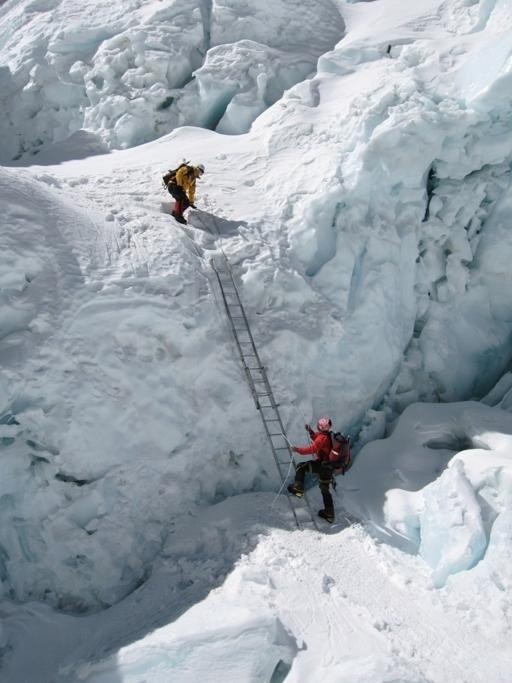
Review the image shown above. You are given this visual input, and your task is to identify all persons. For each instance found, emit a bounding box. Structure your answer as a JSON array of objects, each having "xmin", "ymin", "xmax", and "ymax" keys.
[
  {"xmin": 287, "ymin": 417, "xmax": 335, "ymax": 523},
  {"xmin": 168, "ymin": 163, "xmax": 205, "ymax": 225}
]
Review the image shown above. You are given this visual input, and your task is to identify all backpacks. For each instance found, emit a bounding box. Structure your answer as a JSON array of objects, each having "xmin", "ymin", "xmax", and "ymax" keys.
[
  {"xmin": 163, "ymin": 163, "xmax": 192, "ymax": 185},
  {"xmin": 312, "ymin": 432, "xmax": 350, "ymax": 476}
]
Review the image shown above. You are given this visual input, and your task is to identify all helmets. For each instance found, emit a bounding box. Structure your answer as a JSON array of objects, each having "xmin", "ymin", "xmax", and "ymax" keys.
[
  {"xmin": 317, "ymin": 418, "xmax": 332, "ymax": 431},
  {"xmin": 198, "ymin": 164, "xmax": 205, "ymax": 170}
]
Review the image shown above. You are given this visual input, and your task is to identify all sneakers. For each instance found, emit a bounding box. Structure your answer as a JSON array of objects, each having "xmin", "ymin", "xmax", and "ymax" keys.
[
  {"xmin": 171, "ymin": 210, "xmax": 187, "ymax": 225},
  {"xmin": 319, "ymin": 510, "xmax": 334, "ymax": 523},
  {"xmin": 288, "ymin": 485, "xmax": 304, "ymax": 498}
]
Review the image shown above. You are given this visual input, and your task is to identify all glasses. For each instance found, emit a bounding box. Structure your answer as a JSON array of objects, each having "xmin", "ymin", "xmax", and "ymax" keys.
[{"xmin": 198, "ymin": 167, "xmax": 204, "ymax": 173}]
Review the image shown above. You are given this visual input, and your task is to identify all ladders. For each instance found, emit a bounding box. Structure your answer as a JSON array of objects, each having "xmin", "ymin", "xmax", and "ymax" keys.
[{"xmin": 204, "ymin": 252, "xmax": 321, "ymax": 533}]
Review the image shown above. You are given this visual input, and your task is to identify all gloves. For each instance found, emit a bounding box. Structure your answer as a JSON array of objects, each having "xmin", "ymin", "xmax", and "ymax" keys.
[
  {"xmin": 189, "ymin": 202, "xmax": 194, "ymax": 206},
  {"xmin": 292, "ymin": 447, "xmax": 296, "ymax": 451},
  {"xmin": 177, "ymin": 186, "xmax": 183, "ymax": 190},
  {"xmin": 305, "ymin": 424, "xmax": 311, "ymax": 430}
]
